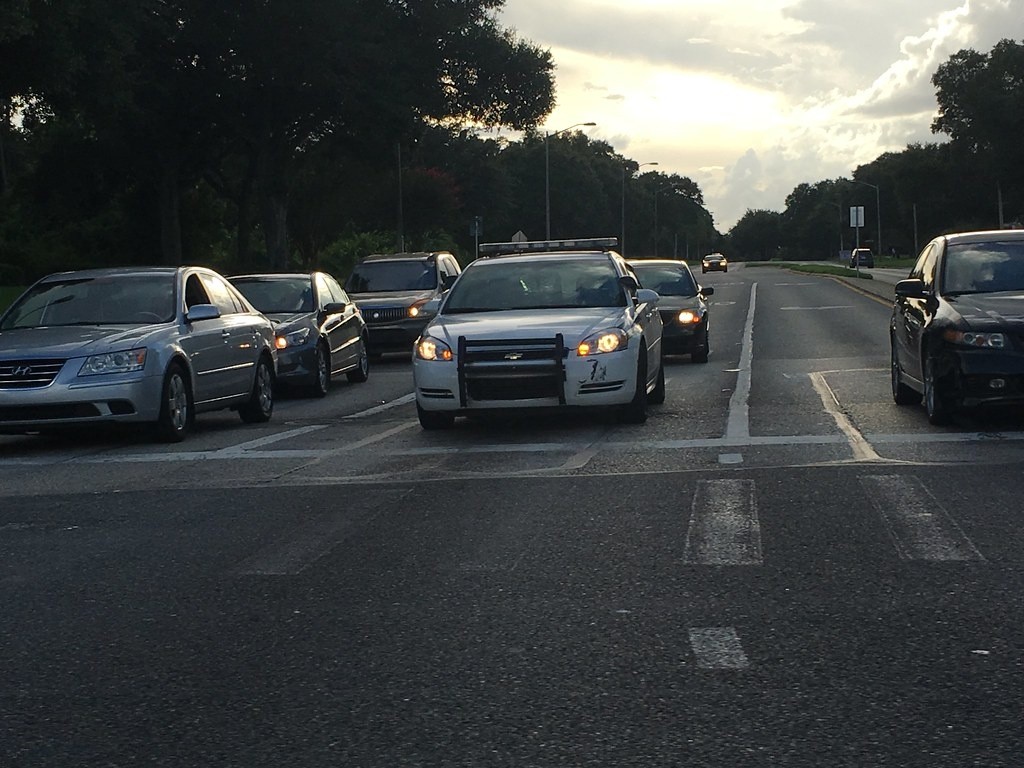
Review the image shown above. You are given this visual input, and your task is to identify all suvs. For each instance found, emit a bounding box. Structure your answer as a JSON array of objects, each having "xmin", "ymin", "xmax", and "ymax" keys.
[{"xmin": 342, "ymin": 251, "xmax": 461, "ymax": 364}]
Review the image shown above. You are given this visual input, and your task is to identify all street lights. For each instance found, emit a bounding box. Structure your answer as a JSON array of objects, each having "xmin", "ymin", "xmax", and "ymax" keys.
[
  {"xmin": 849, "ymin": 180, "xmax": 881, "ymax": 260},
  {"xmin": 621, "ymin": 162, "xmax": 658, "ymax": 255},
  {"xmin": 544, "ymin": 123, "xmax": 597, "ymax": 246}
]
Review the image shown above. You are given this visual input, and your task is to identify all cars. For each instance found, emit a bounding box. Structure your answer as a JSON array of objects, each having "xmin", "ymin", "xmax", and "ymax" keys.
[
  {"xmin": 223, "ymin": 272, "xmax": 370, "ymax": 399},
  {"xmin": 890, "ymin": 229, "xmax": 1024, "ymax": 432},
  {"xmin": 850, "ymin": 248, "xmax": 874, "ymax": 268},
  {"xmin": 702, "ymin": 253, "xmax": 728, "ymax": 273},
  {"xmin": 412, "ymin": 237, "xmax": 664, "ymax": 432},
  {"xmin": 626, "ymin": 259, "xmax": 715, "ymax": 363},
  {"xmin": 0, "ymin": 267, "xmax": 279, "ymax": 457}
]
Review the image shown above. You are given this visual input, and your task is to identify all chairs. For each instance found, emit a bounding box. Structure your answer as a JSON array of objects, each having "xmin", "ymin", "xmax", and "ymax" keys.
[{"xmin": 486, "ymin": 276, "xmax": 530, "ymax": 308}]
